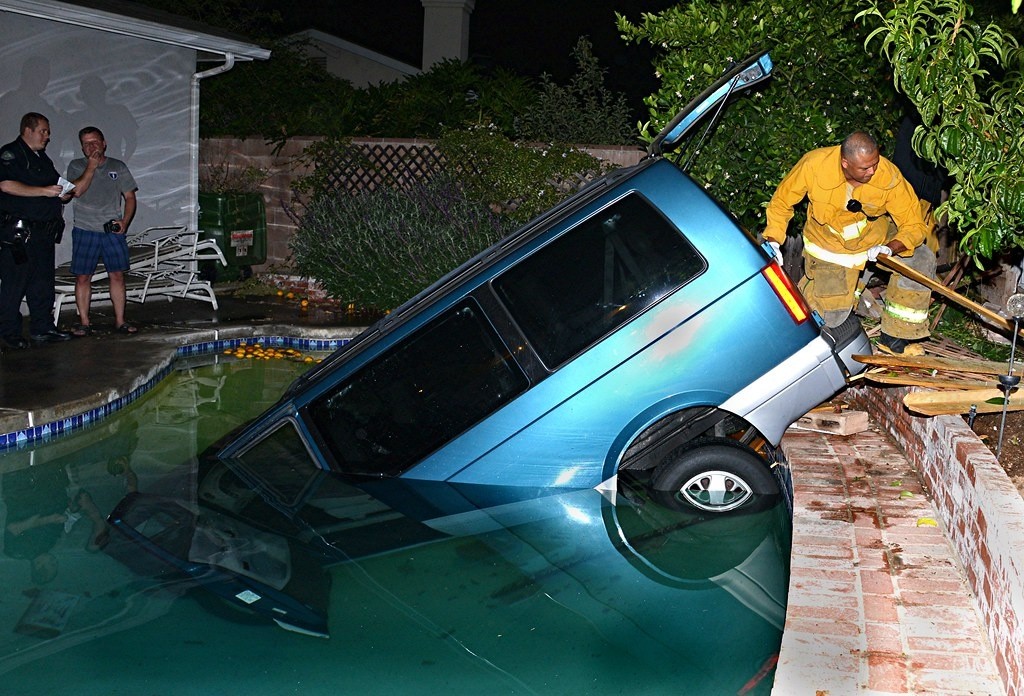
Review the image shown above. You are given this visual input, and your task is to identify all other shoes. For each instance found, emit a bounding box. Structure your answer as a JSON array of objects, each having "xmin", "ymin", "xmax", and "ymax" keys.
[{"xmin": 874, "ymin": 331, "xmax": 925, "ymax": 360}]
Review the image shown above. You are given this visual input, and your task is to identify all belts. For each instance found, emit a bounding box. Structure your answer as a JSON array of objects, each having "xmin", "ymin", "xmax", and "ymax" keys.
[{"xmin": 0, "ymin": 210, "xmax": 46, "ymax": 231}]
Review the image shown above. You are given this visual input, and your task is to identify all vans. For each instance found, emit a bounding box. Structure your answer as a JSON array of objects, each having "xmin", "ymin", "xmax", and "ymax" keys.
[{"xmin": 105, "ymin": 44, "xmax": 872, "ymax": 639}]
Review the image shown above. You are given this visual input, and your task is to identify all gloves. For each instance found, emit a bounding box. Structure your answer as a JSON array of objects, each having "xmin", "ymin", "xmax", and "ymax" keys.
[
  {"xmin": 867, "ymin": 246, "xmax": 892, "ymax": 262},
  {"xmin": 769, "ymin": 241, "xmax": 784, "ymax": 267}
]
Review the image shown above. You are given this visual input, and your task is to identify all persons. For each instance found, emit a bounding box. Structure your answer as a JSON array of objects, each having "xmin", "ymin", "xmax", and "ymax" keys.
[
  {"xmin": 0, "ymin": 113, "xmax": 73, "ymax": 347},
  {"xmin": 762, "ymin": 115, "xmax": 957, "ymax": 351},
  {"xmin": 66, "ymin": 126, "xmax": 138, "ymax": 335}
]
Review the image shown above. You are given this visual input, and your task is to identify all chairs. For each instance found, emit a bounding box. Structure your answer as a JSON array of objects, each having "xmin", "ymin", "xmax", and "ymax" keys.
[{"xmin": 22, "ymin": 224, "xmax": 227, "ymax": 326}]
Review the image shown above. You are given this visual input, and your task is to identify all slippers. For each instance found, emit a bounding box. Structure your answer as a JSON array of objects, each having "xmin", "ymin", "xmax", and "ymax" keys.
[
  {"xmin": 74, "ymin": 325, "xmax": 92, "ymax": 337},
  {"xmin": 114, "ymin": 323, "xmax": 138, "ymax": 334}
]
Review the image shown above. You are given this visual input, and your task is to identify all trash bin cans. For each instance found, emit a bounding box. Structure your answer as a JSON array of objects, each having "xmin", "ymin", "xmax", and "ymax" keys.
[{"xmin": 195, "ymin": 189, "xmax": 267, "ymax": 286}]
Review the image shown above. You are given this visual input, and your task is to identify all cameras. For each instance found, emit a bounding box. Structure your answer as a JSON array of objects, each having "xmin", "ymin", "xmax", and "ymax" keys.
[{"xmin": 103, "ymin": 219, "xmax": 121, "ymax": 233}]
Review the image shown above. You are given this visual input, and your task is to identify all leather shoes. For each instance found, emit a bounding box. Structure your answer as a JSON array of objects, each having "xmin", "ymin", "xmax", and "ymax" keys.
[
  {"xmin": 30, "ymin": 325, "xmax": 73, "ymax": 340},
  {"xmin": 0, "ymin": 334, "xmax": 28, "ymax": 349}
]
